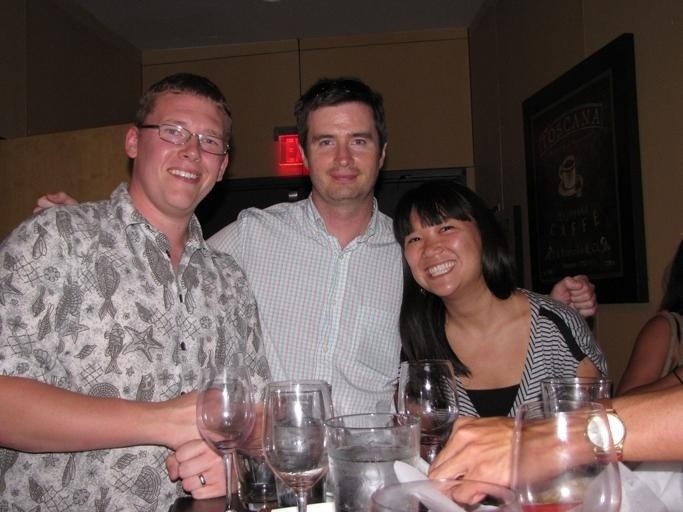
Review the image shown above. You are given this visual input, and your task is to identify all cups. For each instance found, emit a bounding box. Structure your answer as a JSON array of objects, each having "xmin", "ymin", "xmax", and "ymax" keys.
[
  {"xmin": 396, "ymin": 357, "xmax": 459, "ymax": 466},
  {"xmin": 508, "ymin": 402, "xmax": 622, "ymax": 512},
  {"xmin": 322, "ymin": 412, "xmax": 421, "ymax": 512},
  {"xmin": 542, "ymin": 377, "xmax": 614, "ymax": 419},
  {"xmin": 370, "ymin": 477, "xmax": 520, "ymax": 512},
  {"xmin": 193, "ymin": 364, "xmax": 255, "ymax": 511},
  {"xmin": 261, "ymin": 379, "xmax": 333, "ymax": 512},
  {"xmin": 232, "ymin": 445, "xmax": 290, "ymax": 512}
]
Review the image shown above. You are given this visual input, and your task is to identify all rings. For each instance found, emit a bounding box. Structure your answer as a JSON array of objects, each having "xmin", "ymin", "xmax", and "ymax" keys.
[{"xmin": 197, "ymin": 473, "xmax": 207, "ymax": 485}]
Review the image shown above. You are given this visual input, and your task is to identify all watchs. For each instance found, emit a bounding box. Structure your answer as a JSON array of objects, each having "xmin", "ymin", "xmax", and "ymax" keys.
[{"xmin": 586, "ymin": 397, "xmax": 626, "ymax": 464}]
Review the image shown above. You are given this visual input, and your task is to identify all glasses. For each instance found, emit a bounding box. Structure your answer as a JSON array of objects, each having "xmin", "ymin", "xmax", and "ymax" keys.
[{"xmin": 135, "ymin": 124, "xmax": 228, "ymax": 155}]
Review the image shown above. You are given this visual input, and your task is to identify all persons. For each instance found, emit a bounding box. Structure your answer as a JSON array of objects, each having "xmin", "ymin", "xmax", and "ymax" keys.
[
  {"xmin": 32, "ymin": 77, "xmax": 598, "ymax": 429},
  {"xmin": 427, "ymin": 360, "xmax": 683, "ymax": 512},
  {"xmin": 0, "ymin": 72, "xmax": 276, "ymax": 512},
  {"xmin": 393, "ymin": 178, "xmax": 612, "ymax": 451},
  {"xmin": 618, "ymin": 237, "xmax": 683, "ymax": 392}
]
